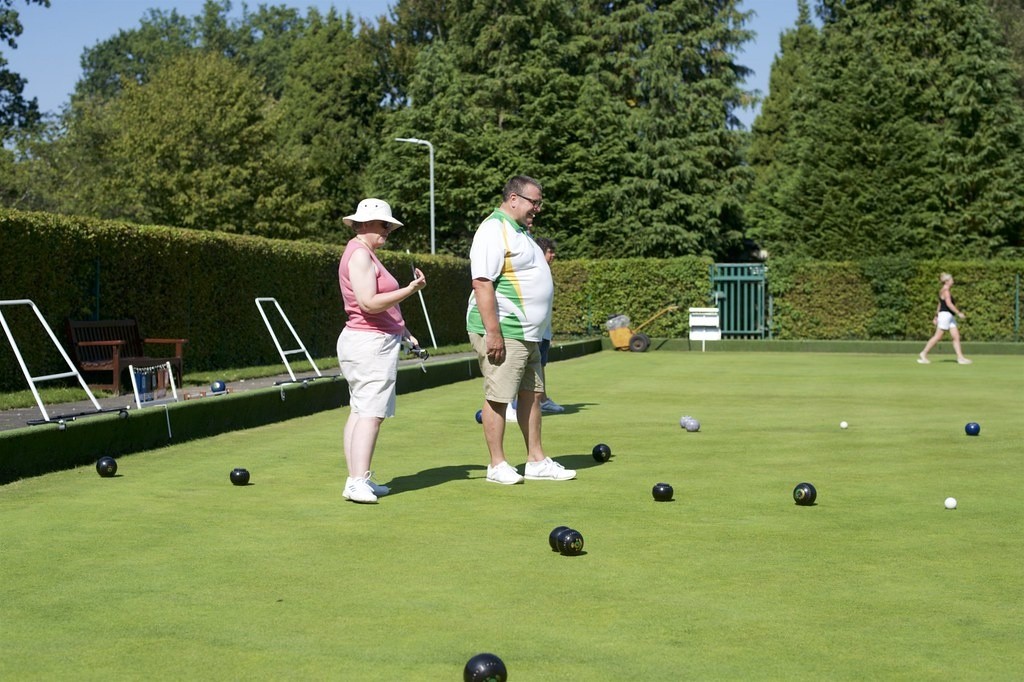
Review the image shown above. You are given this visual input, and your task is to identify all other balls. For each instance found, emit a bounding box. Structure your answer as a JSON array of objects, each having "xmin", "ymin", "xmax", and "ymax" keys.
[
  {"xmin": 840, "ymin": 421, "xmax": 849, "ymax": 428},
  {"xmin": 652, "ymin": 483, "xmax": 674, "ymax": 502},
  {"xmin": 96, "ymin": 457, "xmax": 117, "ymax": 477},
  {"xmin": 592, "ymin": 444, "xmax": 611, "ymax": 463},
  {"xmin": 549, "ymin": 526, "xmax": 570, "ymax": 550},
  {"xmin": 211, "ymin": 381, "xmax": 226, "ymax": 393},
  {"xmin": 230, "ymin": 468, "xmax": 250, "ymax": 486},
  {"xmin": 556, "ymin": 529, "xmax": 585, "ymax": 557},
  {"xmin": 944, "ymin": 497, "xmax": 957, "ymax": 509},
  {"xmin": 685, "ymin": 419, "xmax": 700, "ymax": 431},
  {"xmin": 966, "ymin": 422, "xmax": 980, "ymax": 435},
  {"xmin": 793, "ymin": 482, "xmax": 817, "ymax": 506},
  {"xmin": 475, "ymin": 410, "xmax": 483, "ymax": 424},
  {"xmin": 463, "ymin": 653, "xmax": 507, "ymax": 682},
  {"xmin": 680, "ymin": 416, "xmax": 693, "ymax": 427}
]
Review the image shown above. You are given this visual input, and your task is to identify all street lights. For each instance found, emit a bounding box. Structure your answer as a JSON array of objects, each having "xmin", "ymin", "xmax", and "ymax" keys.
[{"xmin": 393, "ymin": 138, "xmax": 436, "ymax": 257}]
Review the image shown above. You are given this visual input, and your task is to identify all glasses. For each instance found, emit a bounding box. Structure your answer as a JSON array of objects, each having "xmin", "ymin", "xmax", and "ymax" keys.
[
  {"xmin": 373, "ymin": 221, "xmax": 392, "ymax": 229},
  {"xmin": 517, "ymin": 194, "xmax": 544, "ymax": 207}
]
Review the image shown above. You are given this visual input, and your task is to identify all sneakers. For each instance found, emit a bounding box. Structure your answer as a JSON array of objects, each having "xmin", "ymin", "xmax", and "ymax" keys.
[
  {"xmin": 485, "ymin": 461, "xmax": 524, "ymax": 484},
  {"xmin": 523, "ymin": 456, "xmax": 577, "ymax": 481},
  {"xmin": 342, "ymin": 471, "xmax": 389, "ymax": 503}
]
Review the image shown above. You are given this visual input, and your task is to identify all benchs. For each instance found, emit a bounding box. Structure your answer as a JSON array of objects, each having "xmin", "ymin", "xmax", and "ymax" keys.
[{"xmin": 66, "ymin": 318, "xmax": 188, "ymax": 396}]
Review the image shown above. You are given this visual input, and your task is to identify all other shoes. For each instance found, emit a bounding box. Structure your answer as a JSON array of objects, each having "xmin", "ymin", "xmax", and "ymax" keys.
[
  {"xmin": 958, "ymin": 358, "xmax": 972, "ymax": 364},
  {"xmin": 540, "ymin": 398, "xmax": 564, "ymax": 413},
  {"xmin": 506, "ymin": 401, "xmax": 517, "ymax": 423},
  {"xmin": 917, "ymin": 359, "xmax": 931, "ymax": 364}
]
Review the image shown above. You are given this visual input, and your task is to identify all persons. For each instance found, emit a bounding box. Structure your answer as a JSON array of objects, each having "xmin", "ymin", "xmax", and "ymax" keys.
[
  {"xmin": 465, "ymin": 174, "xmax": 576, "ymax": 485},
  {"xmin": 335, "ymin": 198, "xmax": 426, "ymax": 504},
  {"xmin": 503, "ymin": 214, "xmax": 565, "ymax": 423},
  {"xmin": 916, "ymin": 271, "xmax": 973, "ymax": 364}
]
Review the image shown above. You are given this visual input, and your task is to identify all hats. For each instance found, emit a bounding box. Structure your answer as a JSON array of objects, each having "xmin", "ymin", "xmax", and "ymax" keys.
[{"xmin": 341, "ymin": 198, "xmax": 404, "ymax": 231}]
